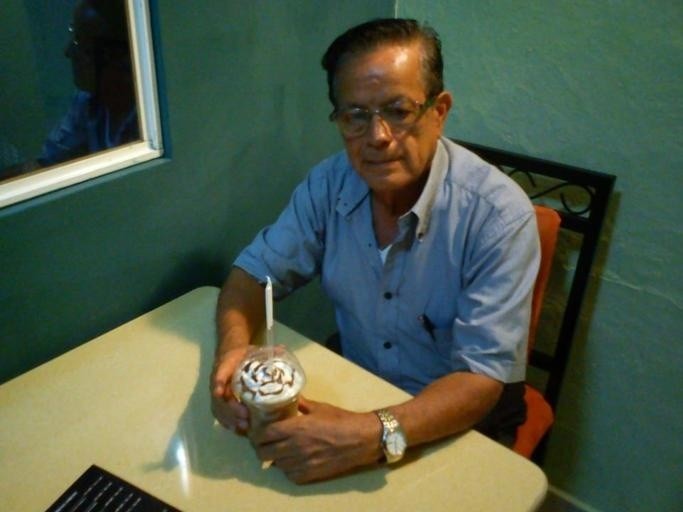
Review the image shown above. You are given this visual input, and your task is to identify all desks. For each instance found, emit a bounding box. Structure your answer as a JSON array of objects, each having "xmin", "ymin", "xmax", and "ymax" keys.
[{"xmin": 2, "ymin": 280, "xmax": 548, "ymax": 511}]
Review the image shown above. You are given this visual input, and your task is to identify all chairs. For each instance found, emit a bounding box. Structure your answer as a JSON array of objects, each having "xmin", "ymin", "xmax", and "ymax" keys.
[{"xmin": 439, "ymin": 141, "xmax": 618, "ymax": 470}]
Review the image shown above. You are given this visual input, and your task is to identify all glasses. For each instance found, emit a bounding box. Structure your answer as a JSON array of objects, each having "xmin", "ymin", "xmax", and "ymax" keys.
[{"xmin": 327, "ymin": 96, "xmax": 434, "ymax": 139}]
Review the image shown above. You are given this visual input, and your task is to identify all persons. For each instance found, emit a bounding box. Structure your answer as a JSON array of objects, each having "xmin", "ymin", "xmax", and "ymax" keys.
[
  {"xmin": 210, "ymin": 19, "xmax": 543, "ymax": 486},
  {"xmin": 20, "ymin": 0, "xmax": 143, "ymax": 180}
]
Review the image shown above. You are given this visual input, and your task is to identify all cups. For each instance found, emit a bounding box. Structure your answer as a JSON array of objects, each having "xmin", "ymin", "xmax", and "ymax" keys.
[{"xmin": 231, "ymin": 346, "xmax": 305, "ymax": 459}]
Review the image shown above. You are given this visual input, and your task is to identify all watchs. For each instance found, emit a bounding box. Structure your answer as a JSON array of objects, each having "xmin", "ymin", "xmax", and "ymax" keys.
[{"xmin": 375, "ymin": 409, "xmax": 406, "ymax": 467}]
